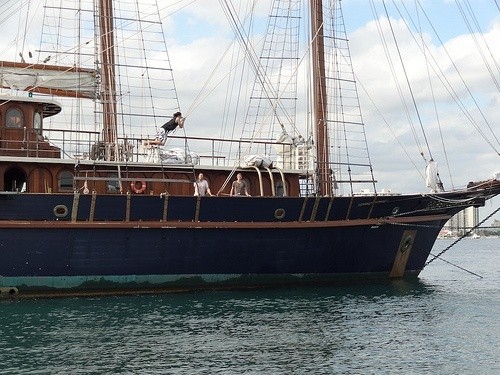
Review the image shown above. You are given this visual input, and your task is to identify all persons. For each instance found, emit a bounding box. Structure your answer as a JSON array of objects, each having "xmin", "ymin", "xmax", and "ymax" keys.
[
  {"xmin": 145, "ymin": 112, "xmax": 186, "ymax": 147},
  {"xmin": 230, "ymin": 173, "xmax": 251, "ymax": 197},
  {"xmin": 194, "ymin": 173, "xmax": 215, "ymax": 196}
]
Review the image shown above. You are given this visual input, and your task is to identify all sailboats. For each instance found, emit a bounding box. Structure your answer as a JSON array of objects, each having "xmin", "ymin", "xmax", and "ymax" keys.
[{"xmin": 0, "ymin": 0, "xmax": 499, "ymax": 299}]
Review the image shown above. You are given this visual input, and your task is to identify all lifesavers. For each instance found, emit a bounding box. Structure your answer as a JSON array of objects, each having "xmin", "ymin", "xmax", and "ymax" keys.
[{"xmin": 130, "ymin": 178, "xmax": 147, "ymax": 194}]
